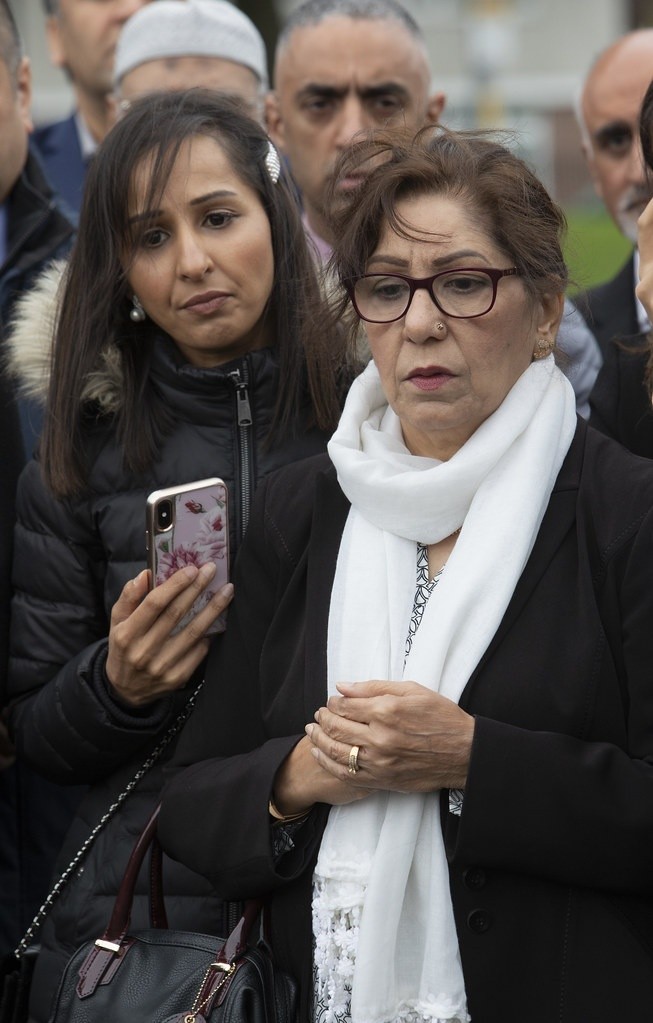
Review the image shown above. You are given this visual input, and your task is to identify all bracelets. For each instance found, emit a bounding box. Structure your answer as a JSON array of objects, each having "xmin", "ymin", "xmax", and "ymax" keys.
[{"xmin": 269, "ymin": 794, "xmax": 312, "ymax": 822}]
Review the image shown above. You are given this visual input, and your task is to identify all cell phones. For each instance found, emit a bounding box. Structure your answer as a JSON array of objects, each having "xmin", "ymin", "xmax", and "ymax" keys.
[{"xmin": 145, "ymin": 477, "xmax": 227, "ymax": 634}]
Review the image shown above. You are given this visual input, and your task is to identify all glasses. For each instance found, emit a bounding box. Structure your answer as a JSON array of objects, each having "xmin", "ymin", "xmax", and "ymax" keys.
[{"xmin": 342, "ymin": 268, "xmax": 545, "ymax": 324}]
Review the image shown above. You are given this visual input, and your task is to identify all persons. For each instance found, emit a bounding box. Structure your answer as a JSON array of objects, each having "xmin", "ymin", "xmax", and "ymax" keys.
[
  {"xmin": 0, "ymin": 0, "xmax": 653, "ymax": 690},
  {"xmin": 0, "ymin": 87, "xmax": 366, "ymax": 1023},
  {"xmin": 156, "ymin": 122, "xmax": 653, "ymax": 1023}
]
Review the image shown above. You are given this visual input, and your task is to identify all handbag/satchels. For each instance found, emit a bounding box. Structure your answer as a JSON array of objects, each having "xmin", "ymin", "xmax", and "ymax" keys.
[
  {"xmin": 0, "ymin": 951, "xmax": 42, "ymax": 1023},
  {"xmin": 48, "ymin": 804, "xmax": 296, "ymax": 1023}
]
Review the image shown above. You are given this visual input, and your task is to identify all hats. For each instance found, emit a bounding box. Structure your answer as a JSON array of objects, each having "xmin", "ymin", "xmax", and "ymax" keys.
[{"xmin": 110, "ymin": 0, "xmax": 267, "ymax": 96}]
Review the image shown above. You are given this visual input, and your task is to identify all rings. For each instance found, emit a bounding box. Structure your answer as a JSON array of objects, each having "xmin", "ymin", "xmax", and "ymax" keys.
[{"xmin": 349, "ymin": 746, "xmax": 361, "ymax": 774}]
